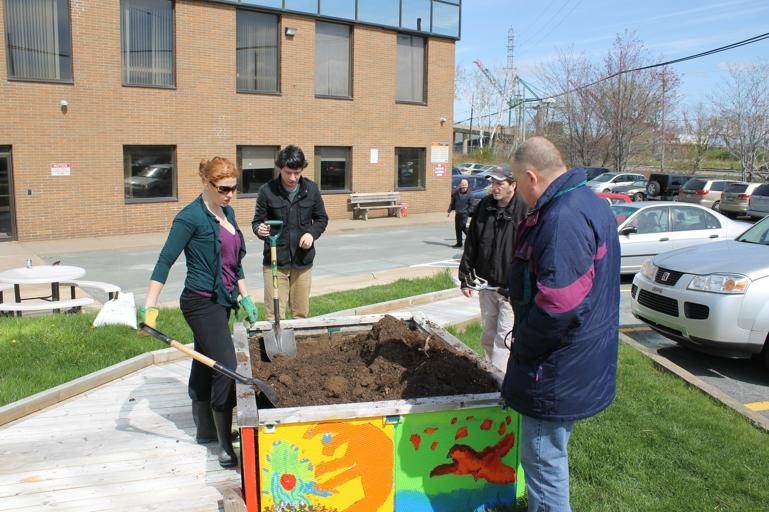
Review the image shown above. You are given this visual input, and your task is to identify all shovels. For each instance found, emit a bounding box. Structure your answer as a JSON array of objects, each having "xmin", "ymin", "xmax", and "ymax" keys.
[
  {"xmin": 261, "ymin": 220, "xmax": 297, "ymax": 362},
  {"xmin": 138, "ymin": 322, "xmax": 279, "ymax": 408}
]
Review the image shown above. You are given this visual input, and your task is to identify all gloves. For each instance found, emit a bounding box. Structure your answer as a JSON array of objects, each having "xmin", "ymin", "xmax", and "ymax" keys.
[
  {"xmin": 136, "ymin": 303, "xmax": 159, "ymax": 337},
  {"xmin": 238, "ymin": 293, "xmax": 259, "ymax": 325}
]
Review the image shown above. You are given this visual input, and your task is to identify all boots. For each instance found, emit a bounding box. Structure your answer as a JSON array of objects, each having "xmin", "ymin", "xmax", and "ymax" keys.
[{"xmin": 195, "ymin": 397, "xmax": 239, "ymax": 468}]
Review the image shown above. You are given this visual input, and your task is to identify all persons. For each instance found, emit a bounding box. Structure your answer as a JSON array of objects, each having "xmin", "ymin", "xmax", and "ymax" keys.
[
  {"xmin": 252, "ymin": 143, "xmax": 328, "ymax": 321},
  {"xmin": 501, "ymin": 135, "xmax": 621, "ymax": 512},
  {"xmin": 447, "ymin": 179, "xmax": 474, "ymax": 247},
  {"xmin": 458, "ymin": 164, "xmax": 529, "ymax": 375},
  {"xmin": 141, "ymin": 156, "xmax": 258, "ymax": 468}
]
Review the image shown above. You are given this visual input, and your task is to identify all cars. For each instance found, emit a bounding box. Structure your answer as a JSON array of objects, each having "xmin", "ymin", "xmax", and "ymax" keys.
[
  {"xmin": 719, "ymin": 183, "xmax": 767, "ymax": 218},
  {"xmin": 123, "ymin": 152, "xmax": 173, "ymax": 199},
  {"xmin": 679, "ymin": 177, "xmax": 748, "ymax": 216},
  {"xmin": 609, "ymin": 201, "xmax": 756, "ymax": 276},
  {"xmin": 746, "ymin": 183, "xmax": 769, "ymax": 221},
  {"xmin": 399, "ymin": 159, "xmax": 418, "ymax": 185},
  {"xmin": 629, "ymin": 214, "xmax": 769, "ymax": 362},
  {"xmin": 452, "ymin": 162, "xmax": 647, "ymax": 230},
  {"xmin": 321, "ymin": 160, "xmax": 344, "ymax": 186}
]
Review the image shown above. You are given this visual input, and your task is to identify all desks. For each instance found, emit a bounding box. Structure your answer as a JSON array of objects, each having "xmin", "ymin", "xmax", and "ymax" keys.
[{"xmin": 0, "ymin": 265, "xmax": 86, "ymax": 317}]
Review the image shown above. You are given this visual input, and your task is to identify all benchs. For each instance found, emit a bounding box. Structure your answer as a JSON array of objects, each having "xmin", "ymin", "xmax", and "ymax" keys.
[
  {"xmin": 59, "ymin": 280, "xmax": 121, "ymax": 309},
  {"xmin": 0, "ymin": 298, "xmax": 94, "ymax": 318},
  {"xmin": 0, "ymin": 283, "xmax": 14, "ymax": 303},
  {"xmin": 350, "ymin": 192, "xmax": 402, "ymax": 220}
]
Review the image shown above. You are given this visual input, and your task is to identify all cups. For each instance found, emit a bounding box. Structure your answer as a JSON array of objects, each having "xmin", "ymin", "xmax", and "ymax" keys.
[{"xmin": 25, "ymin": 259, "xmax": 33, "ymax": 270}]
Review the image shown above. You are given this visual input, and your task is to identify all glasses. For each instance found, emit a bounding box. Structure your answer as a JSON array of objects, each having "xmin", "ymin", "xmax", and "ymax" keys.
[{"xmin": 209, "ymin": 180, "xmax": 238, "ymax": 195}]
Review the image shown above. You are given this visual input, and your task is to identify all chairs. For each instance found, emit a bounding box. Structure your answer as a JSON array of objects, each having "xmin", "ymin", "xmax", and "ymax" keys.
[
  {"xmin": 673, "ymin": 212, "xmax": 710, "ymax": 230},
  {"xmin": 646, "ymin": 212, "xmax": 663, "ymax": 232}
]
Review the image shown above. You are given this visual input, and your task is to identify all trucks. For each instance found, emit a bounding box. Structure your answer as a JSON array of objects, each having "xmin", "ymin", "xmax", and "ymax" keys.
[{"xmin": 671, "ymin": 134, "xmax": 699, "ymax": 145}]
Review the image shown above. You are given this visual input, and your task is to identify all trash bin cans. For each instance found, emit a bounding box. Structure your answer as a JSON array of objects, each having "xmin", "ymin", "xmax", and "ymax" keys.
[{"xmin": 399, "ymin": 203, "xmax": 407, "ymax": 216}]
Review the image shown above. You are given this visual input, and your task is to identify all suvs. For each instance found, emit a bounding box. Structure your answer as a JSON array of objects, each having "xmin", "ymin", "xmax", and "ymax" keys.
[{"xmin": 646, "ymin": 173, "xmax": 693, "ymax": 202}]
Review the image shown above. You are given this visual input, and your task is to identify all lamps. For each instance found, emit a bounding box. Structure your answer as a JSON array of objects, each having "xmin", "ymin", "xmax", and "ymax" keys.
[{"xmin": 285, "ymin": 27, "xmax": 297, "ymax": 36}]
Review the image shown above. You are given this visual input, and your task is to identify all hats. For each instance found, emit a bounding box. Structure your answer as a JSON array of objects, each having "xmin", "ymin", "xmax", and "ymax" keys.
[{"xmin": 484, "ymin": 163, "xmax": 515, "ymax": 182}]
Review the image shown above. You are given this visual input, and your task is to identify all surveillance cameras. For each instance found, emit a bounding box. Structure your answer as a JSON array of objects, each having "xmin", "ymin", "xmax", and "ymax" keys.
[
  {"xmin": 61, "ymin": 100, "xmax": 68, "ymax": 110},
  {"xmin": 440, "ymin": 118, "xmax": 446, "ymax": 123}
]
